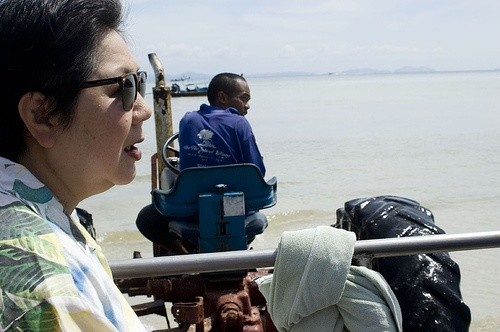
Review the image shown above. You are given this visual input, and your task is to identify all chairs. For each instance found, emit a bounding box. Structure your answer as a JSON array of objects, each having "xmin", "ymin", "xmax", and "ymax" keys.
[{"xmin": 149, "ymin": 163, "xmax": 278, "ymax": 220}]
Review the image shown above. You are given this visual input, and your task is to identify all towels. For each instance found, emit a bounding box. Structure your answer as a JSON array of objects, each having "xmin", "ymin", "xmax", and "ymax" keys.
[{"xmin": 254, "ymin": 224, "xmax": 403, "ymax": 332}]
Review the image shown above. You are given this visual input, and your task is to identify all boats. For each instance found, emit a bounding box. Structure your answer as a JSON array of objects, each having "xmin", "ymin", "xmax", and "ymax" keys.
[{"xmin": 170, "ymin": 82, "xmax": 209, "ymax": 97}]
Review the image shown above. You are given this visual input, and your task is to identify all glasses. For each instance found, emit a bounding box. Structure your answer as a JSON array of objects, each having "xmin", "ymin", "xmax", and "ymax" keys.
[{"xmin": 80, "ymin": 71, "xmax": 147, "ymax": 111}]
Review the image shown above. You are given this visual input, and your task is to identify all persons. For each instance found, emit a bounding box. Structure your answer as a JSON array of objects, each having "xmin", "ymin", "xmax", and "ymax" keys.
[
  {"xmin": 136, "ymin": 73, "xmax": 267, "ymax": 256},
  {"xmin": 0, "ymin": 0, "xmax": 153, "ymax": 332}
]
